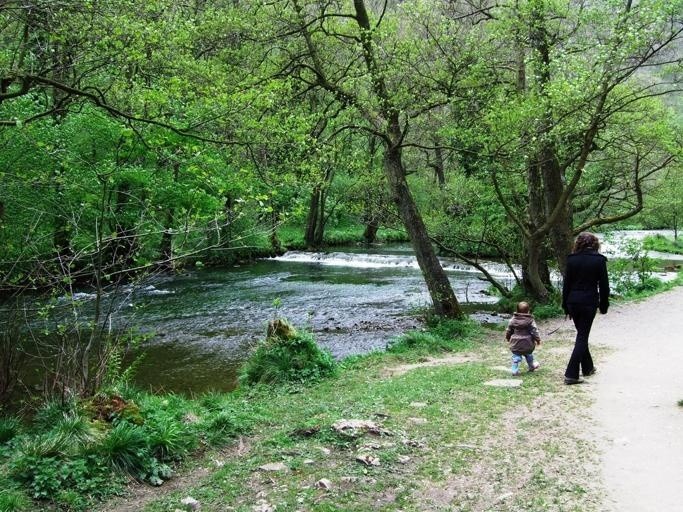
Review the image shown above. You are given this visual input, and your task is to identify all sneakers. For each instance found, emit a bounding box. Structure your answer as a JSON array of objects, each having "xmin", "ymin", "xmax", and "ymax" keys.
[
  {"xmin": 565, "ymin": 377, "xmax": 584, "ymax": 384},
  {"xmin": 530, "ymin": 362, "xmax": 539, "ymax": 371},
  {"xmin": 584, "ymin": 367, "xmax": 596, "ymax": 376},
  {"xmin": 513, "ymin": 370, "xmax": 522, "ymax": 375}
]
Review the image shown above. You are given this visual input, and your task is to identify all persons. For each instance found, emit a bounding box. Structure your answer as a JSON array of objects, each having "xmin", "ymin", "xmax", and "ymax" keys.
[
  {"xmin": 505, "ymin": 301, "xmax": 541, "ymax": 376},
  {"xmin": 562, "ymin": 232, "xmax": 610, "ymax": 385}
]
